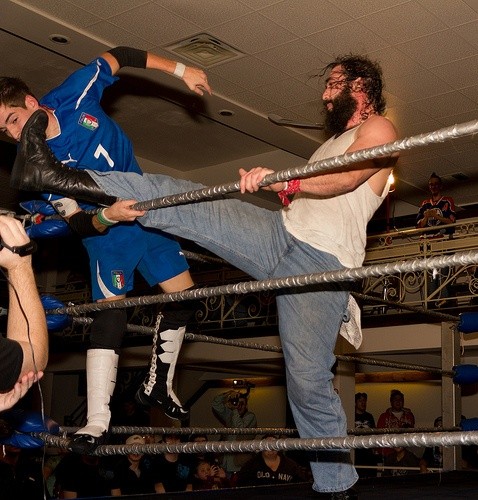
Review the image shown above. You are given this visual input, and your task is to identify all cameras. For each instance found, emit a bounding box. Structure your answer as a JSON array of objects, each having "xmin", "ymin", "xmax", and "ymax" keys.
[{"xmin": 228, "ymin": 396, "xmax": 239, "ymax": 407}]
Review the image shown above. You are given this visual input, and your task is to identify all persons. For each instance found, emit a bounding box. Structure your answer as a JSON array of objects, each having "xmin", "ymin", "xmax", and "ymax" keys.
[
  {"xmin": 9, "ymin": 56, "xmax": 398, "ymax": 500},
  {"xmin": 0, "ymin": 45, "xmax": 212, "ymax": 446},
  {"xmin": 212, "ymin": 390, "xmax": 258, "ymax": 474},
  {"xmin": 416, "ymin": 173, "xmax": 459, "ymax": 307},
  {"xmin": 0, "ymin": 419, "xmax": 228, "ymax": 500},
  {"xmin": 355, "ymin": 392, "xmax": 379, "ymax": 476},
  {"xmin": 236, "ymin": 435, "xmax": 298, "ymax": 487},
  {"xmin": 0, "ymin": 215, "xmax": 48, "ymax": 412},
  {"xmin": 376, "ymin": 389, "xmax": 442, "ymax": 477}
]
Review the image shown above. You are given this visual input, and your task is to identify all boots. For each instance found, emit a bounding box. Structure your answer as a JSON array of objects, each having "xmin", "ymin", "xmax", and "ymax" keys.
[
  {"xmin": 322, "ymin": 487, "xmax": 356, "ymax": 500},
  {"xmin": 9, "ymin": 109, "xmax": 117, "ymax": 206},
  {"xmin": 71, "ymin": 349, "xmax": 119, "ymax": 452},
  {"xmin": 136, "ymin": 313, "xmax": 190, "ymax": 420}
]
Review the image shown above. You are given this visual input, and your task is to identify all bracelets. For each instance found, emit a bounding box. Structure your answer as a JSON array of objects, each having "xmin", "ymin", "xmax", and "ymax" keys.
[
  {"xmin": 278, "ymin": 179, "xmax": 300, "ymax": 207},
  {"xmin": 173, "ymin": 62, "xmax": 185, "ymax": 79},
  {"xmin": 96, "ymin": 208, "xmax": 119, "ymax": 226}
]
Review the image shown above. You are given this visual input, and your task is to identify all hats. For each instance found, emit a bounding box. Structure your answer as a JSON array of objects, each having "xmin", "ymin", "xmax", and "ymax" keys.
[{"xmin": 126, "ymin": 436, "xmax": 145, "ymax": 444}]
